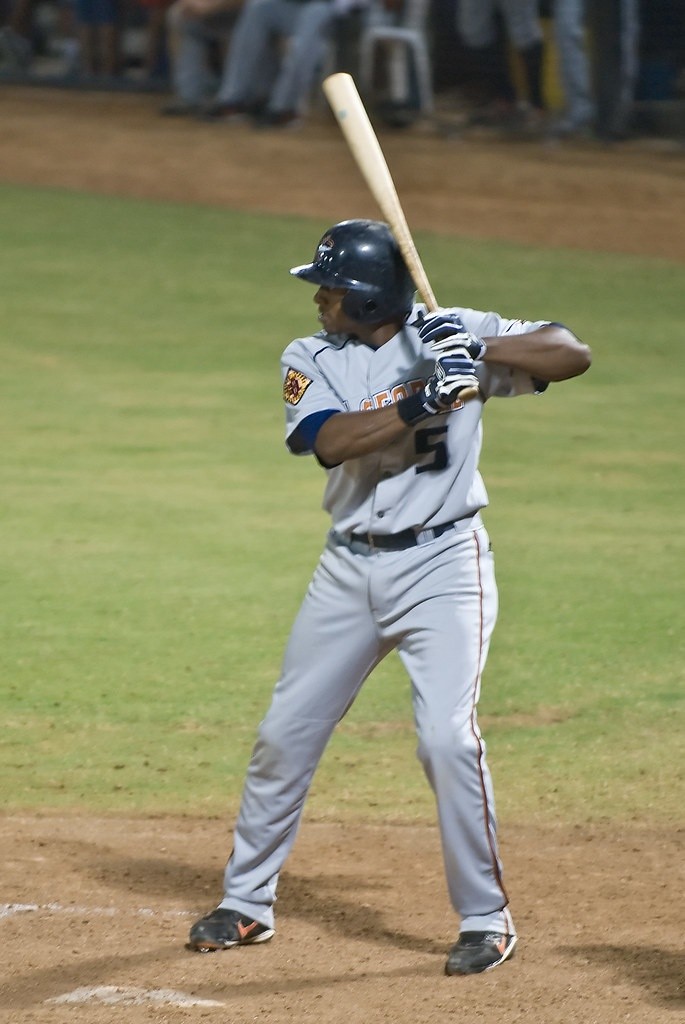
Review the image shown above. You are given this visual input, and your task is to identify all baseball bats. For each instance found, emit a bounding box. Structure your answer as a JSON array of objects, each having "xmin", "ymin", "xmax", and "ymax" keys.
[{"xmin": 321, "ymin": 72, "xmax": 479, "ymax": 402}]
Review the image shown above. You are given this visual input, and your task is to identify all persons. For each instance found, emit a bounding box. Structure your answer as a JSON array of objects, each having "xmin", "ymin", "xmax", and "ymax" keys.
[
  {"xmin": 0, "ymin": 0, "xmax": 642, "ymax": 142},
  {"xmin": 187, "ymin": 218, "xmax": 593, "ymax": 975}
]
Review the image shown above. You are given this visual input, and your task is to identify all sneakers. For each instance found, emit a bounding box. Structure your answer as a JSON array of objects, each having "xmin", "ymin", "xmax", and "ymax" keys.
[
  {"xmin": 189, "ymin": 906, "xmax": 275, "ymax": 952},
  {"xmin": 445, "ymin": 930, "xmax": 519, "ymax": 976}
]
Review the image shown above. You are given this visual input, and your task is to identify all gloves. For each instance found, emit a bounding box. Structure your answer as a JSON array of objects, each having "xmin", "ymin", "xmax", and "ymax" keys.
[
  {"xmin": 411, "ymin": 310, "xmax": 487, "ymax": 362},
  {"xmin": 396, "ymin": 344, "xmax": 480, "ymax": 428}
]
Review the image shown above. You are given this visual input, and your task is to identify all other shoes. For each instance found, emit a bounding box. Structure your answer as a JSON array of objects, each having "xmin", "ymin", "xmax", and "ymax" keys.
[{"xmin": 165, "ymin": 95, "xmax": 297, "ymax": 129}]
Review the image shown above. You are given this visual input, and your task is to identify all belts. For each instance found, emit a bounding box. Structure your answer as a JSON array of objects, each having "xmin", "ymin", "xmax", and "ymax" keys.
[{"xmin": 350, "ymin": 522, "xmax": 454, "ymax": 549}]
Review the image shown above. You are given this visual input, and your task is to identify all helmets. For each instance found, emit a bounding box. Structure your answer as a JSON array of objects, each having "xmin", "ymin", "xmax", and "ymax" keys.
[{"xmin": 289, "ymin": 220, "xmax": 418, "ymax": 324}]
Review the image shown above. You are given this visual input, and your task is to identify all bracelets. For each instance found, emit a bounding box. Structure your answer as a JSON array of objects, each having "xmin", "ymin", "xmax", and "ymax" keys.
[{"xmin": 397, "ymin": 393, "xmax": 432, "ymax": 427}]
